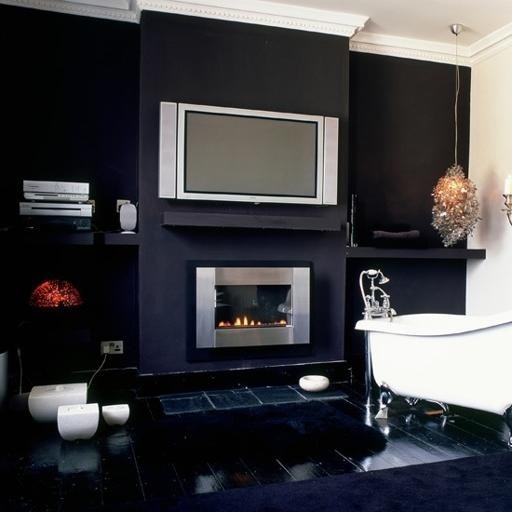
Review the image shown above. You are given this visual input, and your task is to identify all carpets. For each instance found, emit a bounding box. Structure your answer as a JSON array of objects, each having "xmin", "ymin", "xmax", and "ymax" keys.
[{"xmin": 178, "ymin": 448, "xmax": 510, "ymax": 512}]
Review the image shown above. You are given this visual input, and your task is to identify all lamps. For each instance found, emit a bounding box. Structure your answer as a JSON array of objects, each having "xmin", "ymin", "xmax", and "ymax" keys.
[{"xmin": 430, "ymin": 25, "xmax": 484, "ymax": 249}]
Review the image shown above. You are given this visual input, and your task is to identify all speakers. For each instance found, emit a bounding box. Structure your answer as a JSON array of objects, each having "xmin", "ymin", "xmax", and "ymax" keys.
[
  {"xmin": 158, "ymin": 102, "xmax": 178, "ymax": 198},
  {"xmin": 323, "ymin": 116, "xmax": 339, "ymax": 205},
  {"xmin": 119, "ymin": 204, "xmax": 138, "ymax": 234}
]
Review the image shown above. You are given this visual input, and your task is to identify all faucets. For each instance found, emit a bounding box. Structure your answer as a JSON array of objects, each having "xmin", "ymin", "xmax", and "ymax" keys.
[{"xmin": 363, "ymin": 294, "xmax": 399, "ymax": 318}]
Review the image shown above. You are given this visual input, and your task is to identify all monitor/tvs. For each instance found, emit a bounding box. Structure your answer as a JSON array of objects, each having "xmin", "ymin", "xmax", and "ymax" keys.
[{"xmin": 177, "ymin": 101, "xmax": 324, "ymax": 205}]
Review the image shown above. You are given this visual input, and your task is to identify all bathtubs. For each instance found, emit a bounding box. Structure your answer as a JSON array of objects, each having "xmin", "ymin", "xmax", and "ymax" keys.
[{"xmin": 353, "ymin": 313, "xmax": 512, "ymax": 417}]
